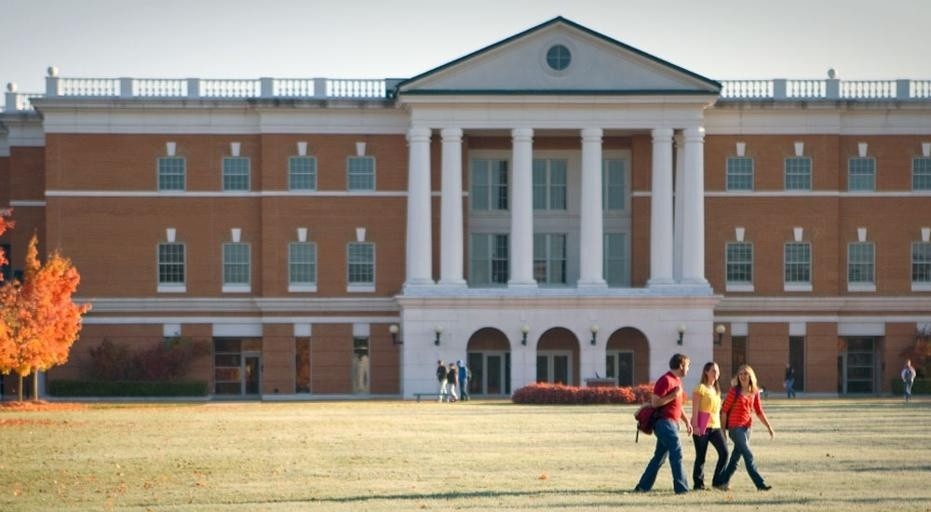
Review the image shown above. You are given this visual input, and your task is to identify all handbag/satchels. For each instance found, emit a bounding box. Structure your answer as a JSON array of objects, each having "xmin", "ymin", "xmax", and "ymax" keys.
[{"xmin": 634, "ymin": 406, "xmax": 661, "ymax": 443}]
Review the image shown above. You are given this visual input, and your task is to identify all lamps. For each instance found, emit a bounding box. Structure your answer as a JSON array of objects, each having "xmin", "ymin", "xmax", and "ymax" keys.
[
  {"xmin": 387, "ymin": 322, "xmax": 403, "ymax": 344},
  {"xmin": 433, "ymin": 327, "xmax": 442, "ymax": 345},
  {"xmin": 520, "ymin": 325, "xmax": 530, "ymax": 346},
  {"xmin": 677, "ymin": 324, "xmax": 686, "ymax": 345},
  {"xmin": 714, "ymin": 325, "xmax": 727, "ymax": 347},
  {"xmin": 589, "ymin": 324, "xmax": 598, "ymax": 344}
]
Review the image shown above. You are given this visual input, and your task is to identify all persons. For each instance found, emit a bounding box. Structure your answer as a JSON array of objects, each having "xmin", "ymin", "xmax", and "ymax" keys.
[
  {"xmin": 436, "ymin": 360, "xmax": 449, "ymax": 403},
  {"xmin": 901, "ymin": 359, "xmax": 916, "ymax": 401},
  {"xmin": 447, "ymin": 362, "xmax": 458, "ymax": 401},
  {"xmin": 783, "ymin": 363, "xmax": 796, "ymax": 399},
  {"xmin": 632, "ymin": 354, "xmax": 694, "ymax": 495},
  {"xmin": 690, "ymin": 362, "xmax": 730, "ymax": 491},
  {"xmin": 457, "ymin": 361, "xmax": 470, "ymax": 401},
  {"xmin": 717, "ymin": 364, "xmax": 773, "ymax": 490}
]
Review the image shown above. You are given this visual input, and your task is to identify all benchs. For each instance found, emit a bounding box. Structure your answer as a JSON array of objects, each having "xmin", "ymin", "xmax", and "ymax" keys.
[{"xmin": 413, "ymin": 392, "xmax": 452, "ymax": 402}]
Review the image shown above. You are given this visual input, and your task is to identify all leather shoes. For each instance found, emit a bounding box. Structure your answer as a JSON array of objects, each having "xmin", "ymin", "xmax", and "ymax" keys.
[{"xmin": 756, "ymin": 482, "xmax": 772, "ymax": 491}]
